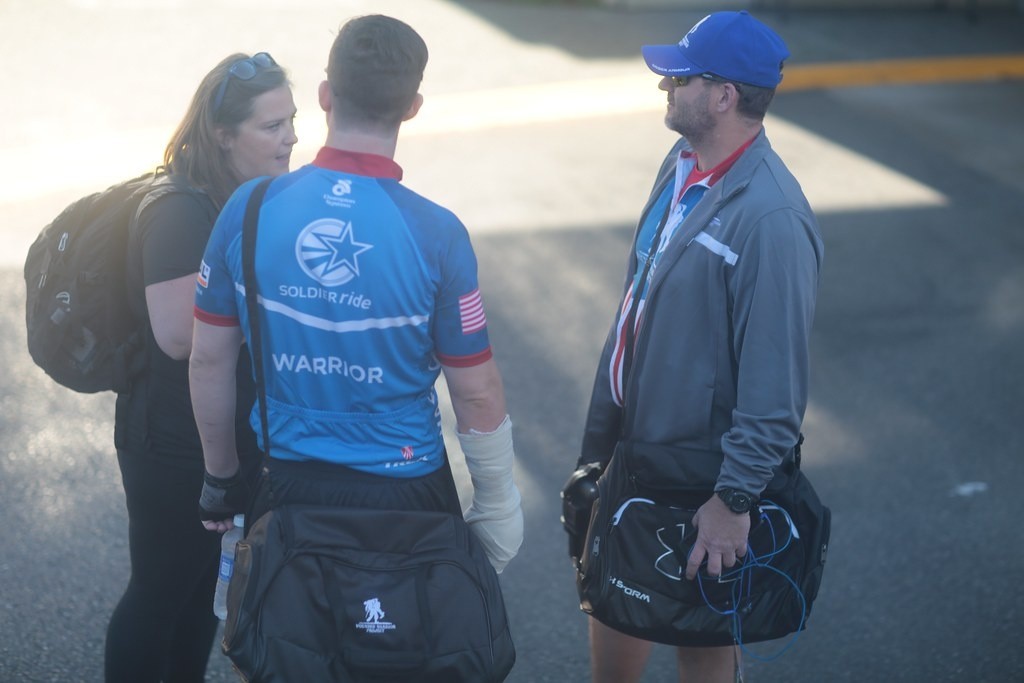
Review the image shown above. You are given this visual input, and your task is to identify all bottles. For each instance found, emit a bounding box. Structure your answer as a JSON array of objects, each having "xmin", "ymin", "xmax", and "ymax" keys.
[{"xmin": 211, "ymin": 513, "xmax": 246, "ymax": 621}]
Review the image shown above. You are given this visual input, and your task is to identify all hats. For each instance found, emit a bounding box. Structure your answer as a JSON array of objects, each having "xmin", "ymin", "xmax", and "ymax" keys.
[{"xmin": 640, "ymin": 10, "xmax": 789, "ymax": 90}]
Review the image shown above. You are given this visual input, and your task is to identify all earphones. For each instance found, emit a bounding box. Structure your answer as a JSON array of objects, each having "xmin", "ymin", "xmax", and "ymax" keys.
[{"xmin": 740, "ymin": 601, "xmax": 754, "ymax": 615}]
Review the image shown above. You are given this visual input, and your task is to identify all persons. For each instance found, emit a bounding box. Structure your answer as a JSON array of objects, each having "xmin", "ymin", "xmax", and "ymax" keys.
[
  {"xmin": 563, "ymin": 11, "xmax": 832, "ymax": 681},
  {"xmin": 105, "ymin": 55, "xmax": 297, "ymax": 683},
  {"xmin": 188, "ymin": 13, "xmax": 528, "ymax": 683}
]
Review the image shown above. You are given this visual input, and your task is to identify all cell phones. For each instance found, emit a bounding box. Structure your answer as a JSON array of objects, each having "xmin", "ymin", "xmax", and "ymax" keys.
[{"xmin": 675, "ymin": 527, "xmax": 708, "ymax": 573}]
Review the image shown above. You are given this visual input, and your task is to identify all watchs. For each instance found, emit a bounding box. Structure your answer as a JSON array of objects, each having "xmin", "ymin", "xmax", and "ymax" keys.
[{"xmin": 717, "ymin": 487, "xmax": 758, "ymax": 513}]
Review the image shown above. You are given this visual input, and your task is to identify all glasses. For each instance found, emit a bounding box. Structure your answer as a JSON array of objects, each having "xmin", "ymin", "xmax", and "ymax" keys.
[
  {"xmin": 670, "ymin": 73, "xmax": 742, "ymax": 92},
  {"xmin": 211, "ymin": 52, "xmax": 276, "ymax": 117}
]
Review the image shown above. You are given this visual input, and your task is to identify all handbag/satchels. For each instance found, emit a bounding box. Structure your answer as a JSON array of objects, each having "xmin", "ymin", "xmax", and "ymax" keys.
[
  {"xmin": 219, "ymin": 502, "xmax": 517, "ymax": 683},
  {"xmin": 560, "ymin": 441, "xmax": 833, "ymax": 649}
]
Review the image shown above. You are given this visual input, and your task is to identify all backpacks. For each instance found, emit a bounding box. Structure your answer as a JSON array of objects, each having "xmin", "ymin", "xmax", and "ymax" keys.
[{"xmin": 24, "ymin": 171, "xmax": 154, "ymax": 394}]
